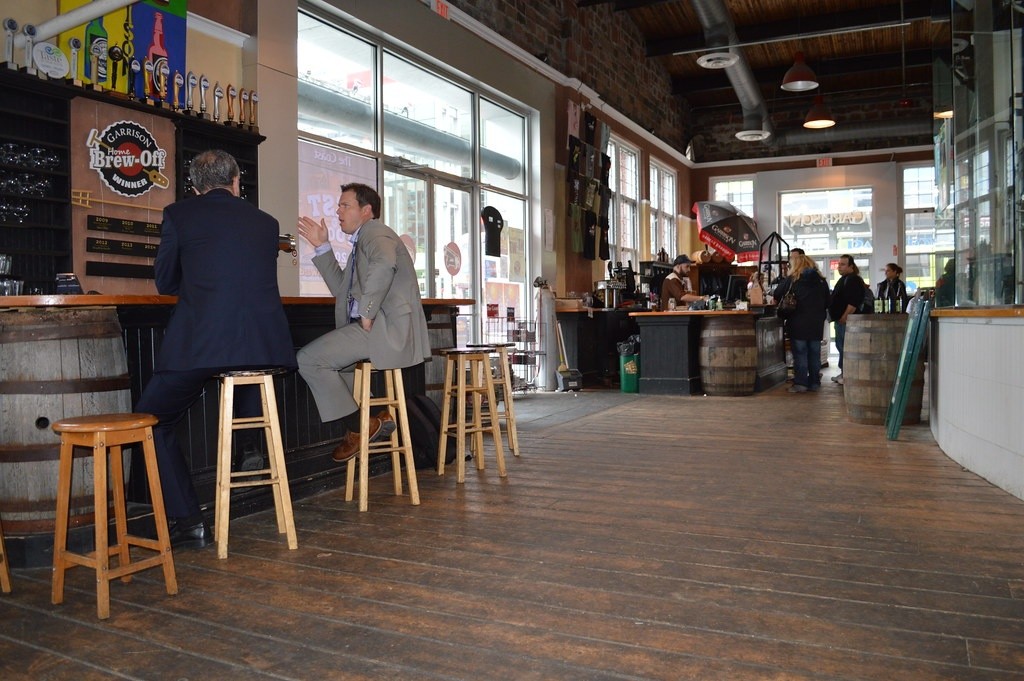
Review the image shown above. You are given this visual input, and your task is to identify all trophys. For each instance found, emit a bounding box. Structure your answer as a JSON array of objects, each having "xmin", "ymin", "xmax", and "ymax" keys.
[{"xmin": 0, "ymin": 18, "xmax": 259, "ymax": 134}]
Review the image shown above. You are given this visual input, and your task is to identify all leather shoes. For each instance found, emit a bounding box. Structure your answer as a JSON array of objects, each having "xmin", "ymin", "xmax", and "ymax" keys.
[
  {"xmin": 231, "ymin": 449, "xmax": 263, "ymax": 493},
  {"xmin": 150, "ymin": 518, "xmax": 214, "ymax": 550}
]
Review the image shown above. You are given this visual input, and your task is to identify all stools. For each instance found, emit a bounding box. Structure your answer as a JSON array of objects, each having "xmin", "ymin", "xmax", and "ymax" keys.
[
  {"xmin": 344, "ymin": 357, "xmax": 421, "ymax": 512},
  {"xmin": 466, "ymin": 342, "xmax": 519, "ymax": 460},
  {"xmin": 51, "ymin": 412, "xmax": 177, "ymax": 621},
  {"xmin": 212, "ymin": 363, "xmax": 298, "ymax": 561},
  {"xmin": 436, "ymin": 347, "xmax": 507, "ymax": 485}
]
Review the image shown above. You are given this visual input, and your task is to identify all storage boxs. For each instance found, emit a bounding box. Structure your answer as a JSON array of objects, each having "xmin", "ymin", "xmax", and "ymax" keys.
[{"xmin": 555, "ymin": 297, "xmax": 583, "ymax": 309}]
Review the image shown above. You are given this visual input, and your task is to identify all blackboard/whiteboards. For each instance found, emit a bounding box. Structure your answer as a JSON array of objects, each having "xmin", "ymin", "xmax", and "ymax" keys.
[{"xmin": 890, "ymin": 300, "xmax": 931, "ymax": 411}]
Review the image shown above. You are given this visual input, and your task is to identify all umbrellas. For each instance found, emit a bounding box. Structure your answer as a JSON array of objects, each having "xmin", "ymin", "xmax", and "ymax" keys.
[{"xmin": 692, "ymin": 200, "xmax": 764, "ymax": 263}]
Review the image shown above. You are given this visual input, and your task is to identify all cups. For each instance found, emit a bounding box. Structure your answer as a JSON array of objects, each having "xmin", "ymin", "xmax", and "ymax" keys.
[
  {"xmin": 0, "ymin": 253, "xmax": 12, "ymax": 274},
  {"xmin": 0, "ymin": 278, "xmax": 24, "ymax": 296}
]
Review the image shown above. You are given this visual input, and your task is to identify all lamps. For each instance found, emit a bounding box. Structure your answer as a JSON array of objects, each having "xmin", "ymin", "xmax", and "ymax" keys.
[
  {"xmin": 802, "ymin": 59, "xmax": 836, "ymax": 129},
  {"xmin": 780, "ymin": 39, "xmax": 819, "ymax": 92}
]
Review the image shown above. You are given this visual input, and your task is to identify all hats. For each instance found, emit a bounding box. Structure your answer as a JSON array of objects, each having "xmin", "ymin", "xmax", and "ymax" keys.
[{"xmin": 672, "ymin": 254, "xmax": 696, "ymax": 266}]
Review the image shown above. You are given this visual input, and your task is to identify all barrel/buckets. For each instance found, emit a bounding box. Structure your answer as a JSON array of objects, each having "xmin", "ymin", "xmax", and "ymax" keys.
[
  {"xmin": 0, "ymin": 309, "xmax": 134, "ymax": 535},
  {"xmin": 843, "ymin": 313, "xmax": 925, "ymax": 425},
  {"xmin": 700, "ymin": 318, "xmax": 757, "ymax": 396}
]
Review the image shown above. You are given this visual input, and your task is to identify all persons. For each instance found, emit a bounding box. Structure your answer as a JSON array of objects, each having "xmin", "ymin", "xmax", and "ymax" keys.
[
  {"xmin": 935, "ymin": 259, "xmax": 955, "ymax": 307},
  {"xmin": 878, "ymin": 263, "xmax": 907, "ymax": 300},
  {"xmin": 771, "ymin": 248, "xmax": 830, "ymax": 393},
  {"xmin": 134, "ymin": 151, "xmax": 300, "ymax": 550},
  {"xmin": 660, "ymin": 254, "xmax": 709, "ymax": 312},
  {"xmin": 297, "ymin": 183, "xmax": 432, "ymax": 462},
  {"xmin": 828, "ymin": 255, "xmax": 875, "ymax": 385}
]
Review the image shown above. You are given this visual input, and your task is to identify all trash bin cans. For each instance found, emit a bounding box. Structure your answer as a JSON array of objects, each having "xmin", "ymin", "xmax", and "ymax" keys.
[
  {"xmin": 556, "ymin": 369, "xmax": 583, "ymax": 391},
  {"xmin": 619, "ymin": 336, "xmax": 640, "ymax": 393}
]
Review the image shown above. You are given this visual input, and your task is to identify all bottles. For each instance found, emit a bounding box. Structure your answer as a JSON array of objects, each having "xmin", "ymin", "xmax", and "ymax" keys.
[
  {"xmin": 884, "ymin": 282, "xmax": 896, "ymax": 313},
  {"xmin": 895, "ymin": 283, "xmax": 906, "ymax": 313},
  {"xmin": 874, "ymin": 283, "xmax": 884, "ymax": 313},
  {"xmin": 668, "ymin": 298, "xmax": 676, "ymax": 311}
]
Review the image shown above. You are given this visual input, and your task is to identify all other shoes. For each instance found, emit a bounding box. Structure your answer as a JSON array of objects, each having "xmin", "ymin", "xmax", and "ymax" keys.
[
  {"xmin": 784, "ymin": 385, "xmax": 806, "ymax": 392},
  {"xmin": 369, "ymin": 411, "xmax": 396, "ymax": 449},
  {"xmin": 837, "ymin": 377, "xmax": 843, "ymax": 384},
  {"xmin": 807, "ymin": 384, "xmax": 819, "ymax": 391},
  {"xmin": 332, "ymin": 417, "xmax": 383, "ymax": 463},
  {"xmin": 831, "ymin": 373, "xmax": 842, "ymax": 381}
]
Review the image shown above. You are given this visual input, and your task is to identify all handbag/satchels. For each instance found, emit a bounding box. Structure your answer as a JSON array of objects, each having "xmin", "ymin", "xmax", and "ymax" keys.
[{"xmin": 778, "ymin": 289, "xmax": 798, "ymax": 319}]
